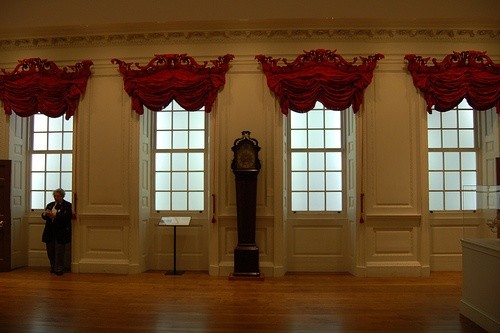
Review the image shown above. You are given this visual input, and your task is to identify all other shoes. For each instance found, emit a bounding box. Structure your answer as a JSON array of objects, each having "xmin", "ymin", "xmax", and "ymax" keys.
[{"xmin": 50, "ymin": 265, "xmax": 63, "ymax": 276}]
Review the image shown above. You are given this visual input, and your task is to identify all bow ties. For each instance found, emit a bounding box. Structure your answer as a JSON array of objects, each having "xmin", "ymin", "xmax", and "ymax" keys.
[{"xmin": 55, "ymin": 203, "xmax": 61, "ymax": 210}]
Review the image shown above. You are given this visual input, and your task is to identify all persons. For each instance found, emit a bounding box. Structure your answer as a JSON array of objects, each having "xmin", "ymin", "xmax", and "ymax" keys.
[{"xmin": 41, "ymin": 189, "xmax": 72, "ymax": 276}]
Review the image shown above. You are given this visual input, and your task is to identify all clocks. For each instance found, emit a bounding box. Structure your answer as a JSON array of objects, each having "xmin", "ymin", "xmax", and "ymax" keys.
[{"xmin": 230, "ymin": 130, "xmax": 262, "ymax": 170}]
